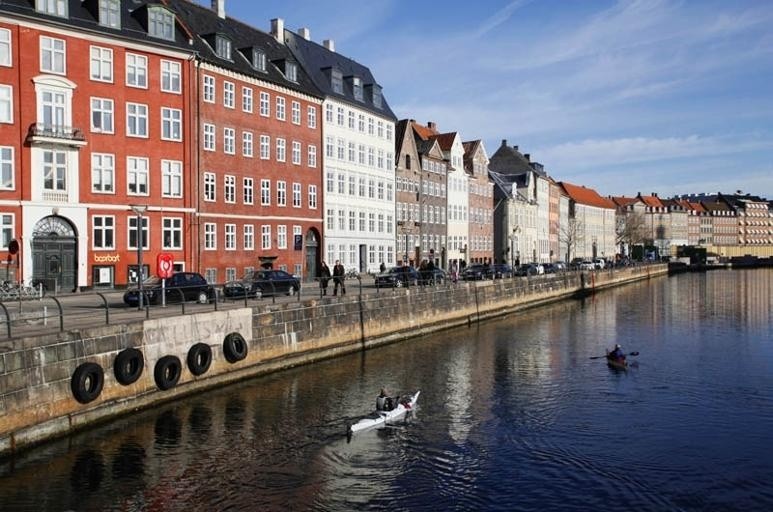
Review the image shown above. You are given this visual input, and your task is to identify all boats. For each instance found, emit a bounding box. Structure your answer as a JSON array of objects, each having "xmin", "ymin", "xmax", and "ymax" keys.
[
  {"xmin": 606, "ymin": 348, "xmax": 630, "ymax": 372},
  {"xmin": 349, "ymin": 390, "xmax": 421, "ymax": 433}
]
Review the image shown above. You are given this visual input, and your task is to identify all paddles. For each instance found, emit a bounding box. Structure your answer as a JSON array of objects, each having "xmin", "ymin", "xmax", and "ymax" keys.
[{"xmin": 590, "ymin": 352, "xmax": 640, "ymax": 359}]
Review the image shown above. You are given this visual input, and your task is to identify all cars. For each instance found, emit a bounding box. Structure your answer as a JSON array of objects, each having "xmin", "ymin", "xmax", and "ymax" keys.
[
  {"xmin": 123, "ymin": 271, "xmax": 211, "ymax": 306},
  {"xmin": 415, "ymin": 266, "xmax": 446, "ymax": 287},
  {"xmin": 375, "ymin": 265, "xmax": 420, "ymax": 288},
  {"xmin": 463, "ymin": 257, "xmax": 635, "ymax": 282},
  {"xmin": 223, "ymin": 270, "xmax": 301, "ymax": 300}
]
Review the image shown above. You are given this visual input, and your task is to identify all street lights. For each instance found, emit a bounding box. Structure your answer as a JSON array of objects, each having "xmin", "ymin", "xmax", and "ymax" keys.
[
  {"xmin": 130, "ymin": 203, "xmax": 149, "ymax": 311},
  {"xmin": 509, "ymin": 235, "xmax": 517, "ymax": 277},
  {"xmin": 400, "ymin": 227, "xmax": 414, "ymax": 288}
]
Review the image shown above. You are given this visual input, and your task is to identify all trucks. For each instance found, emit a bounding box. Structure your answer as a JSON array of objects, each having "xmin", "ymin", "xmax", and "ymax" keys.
[{"xmin": 642, "ymin": 238, "xmax": 688, "ymax": 263}]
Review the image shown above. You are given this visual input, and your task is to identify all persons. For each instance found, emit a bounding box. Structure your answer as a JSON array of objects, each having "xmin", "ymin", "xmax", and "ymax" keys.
[
  {"xmin": 375, "ymin": 385, "xmax": 399, "ymax": 411},
  {"xmin": 426, "ymin": 260, "xmax": 436, "ymax": 287},
  {"xmin": 482, "ymin": 258, "xmax": 490, "ymax": 280},
  {"xmin": 610, "ymin": 343, "xmax": 627, "ymax": 364},
  {"xmin": 514, "ymin": 256, "xmax": 520, "ymax": 270},
  {"xmin": 379, "ymin": 263, "xmax": 386, "ymax": 272},
  {"xmin": 332, "ymin": 260, "xmax": 346, "ymax": 297},
  {"xmin": 314, "ymin": 261, "xmax": 331, "ymax": 296},
  {"xmin": 418, "ymin": 260, "xmax": 428, "ymax": 288}
]
